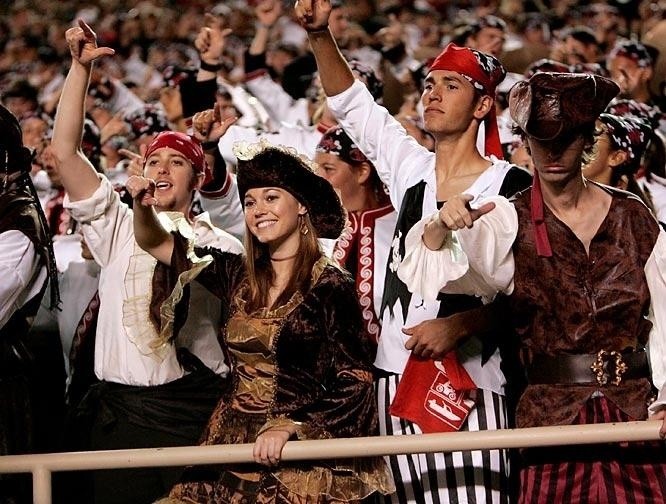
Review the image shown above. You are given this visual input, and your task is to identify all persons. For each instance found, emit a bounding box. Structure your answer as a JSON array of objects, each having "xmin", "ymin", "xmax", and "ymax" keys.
[{"xmin": 1, "ymin": 1, "xmax": 666, "ymax": 504}]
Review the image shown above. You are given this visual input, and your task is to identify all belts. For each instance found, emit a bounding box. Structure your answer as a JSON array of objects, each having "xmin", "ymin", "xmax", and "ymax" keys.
[{"xmin": 520, "ymin": 349, "xmax": 652, "ymax": 385}]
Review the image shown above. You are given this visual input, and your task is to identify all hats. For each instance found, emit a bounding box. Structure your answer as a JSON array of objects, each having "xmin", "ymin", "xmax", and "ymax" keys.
[
  {"xmin": 506, "ymin": 70, "xmax": 620, "ymax": 141},
  {"xmin": 232, "ymin": 140, "xmax": 345, "ymax": 239},
  {"xmin": 0, "ymin": 80, "xmax": 39, "ymax": 112}
]
